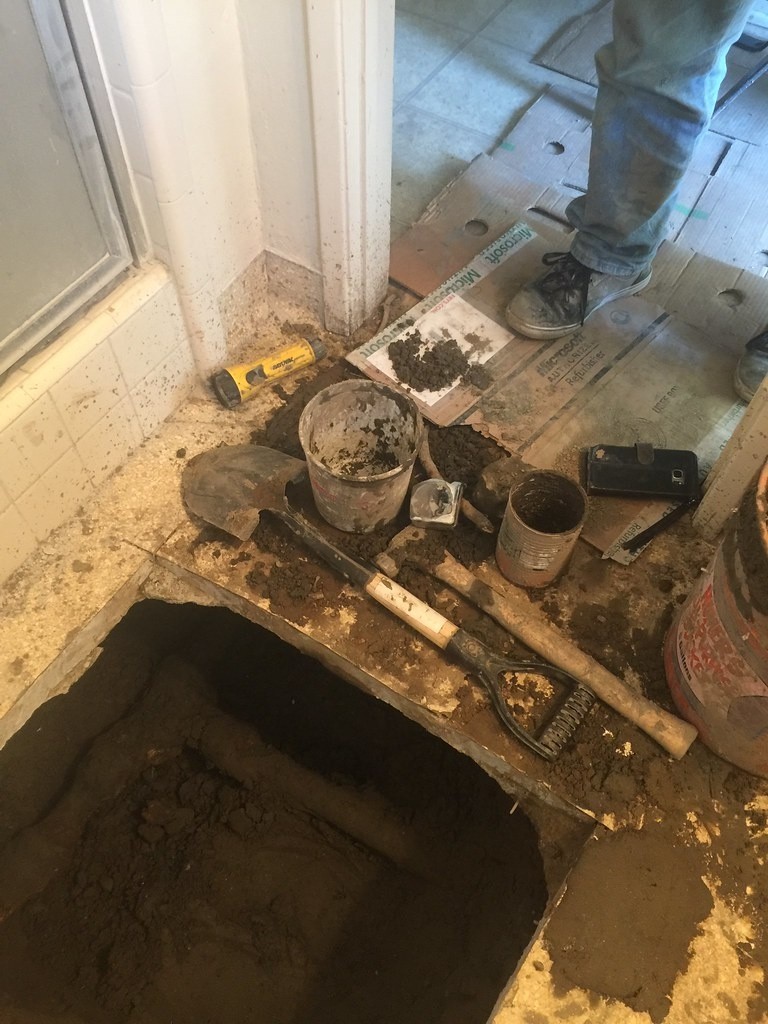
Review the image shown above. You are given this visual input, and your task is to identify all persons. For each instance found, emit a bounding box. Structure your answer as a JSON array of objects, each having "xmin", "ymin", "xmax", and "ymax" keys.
[{"xmin": 506, "ymin": 0, "xmax": 766, "ymax": 404}]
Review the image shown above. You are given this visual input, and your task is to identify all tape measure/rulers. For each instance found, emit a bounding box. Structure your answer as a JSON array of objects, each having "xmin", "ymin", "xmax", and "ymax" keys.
[{"xmin": 408, "ymin": 479, "xmax": 467, "ymax": 528}]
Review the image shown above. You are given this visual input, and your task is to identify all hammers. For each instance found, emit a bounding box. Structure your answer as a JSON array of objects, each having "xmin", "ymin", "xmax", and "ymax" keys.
[{"xmin": 372, "ymin": 523, "xmax": 700, "ymax": 760}]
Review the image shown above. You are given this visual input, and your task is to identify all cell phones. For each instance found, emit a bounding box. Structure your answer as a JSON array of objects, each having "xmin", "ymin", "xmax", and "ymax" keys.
[{"xmin": 587, "ymin": 444, "xmax": 702, "ymax": 502}]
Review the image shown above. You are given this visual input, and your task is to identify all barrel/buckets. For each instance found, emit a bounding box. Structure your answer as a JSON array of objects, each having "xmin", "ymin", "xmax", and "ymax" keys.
[
  {"xmin": 298, "ymin": 378, "xmax": 426, "ymax": 534},
  {"xmin": 493, "ymin": 468, "xmax": 590, "ymax": 586}
]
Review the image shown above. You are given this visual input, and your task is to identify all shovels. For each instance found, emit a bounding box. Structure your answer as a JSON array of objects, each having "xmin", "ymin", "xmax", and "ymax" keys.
[{"xmin": 180, "ymin": 443, "xmax": 596, "ymax": 763}]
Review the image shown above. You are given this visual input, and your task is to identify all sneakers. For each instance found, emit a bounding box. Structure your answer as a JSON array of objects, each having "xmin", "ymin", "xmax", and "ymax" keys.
[{"xmin": 502, "ymin": 252, "xmax": 653, "ymax": 340}]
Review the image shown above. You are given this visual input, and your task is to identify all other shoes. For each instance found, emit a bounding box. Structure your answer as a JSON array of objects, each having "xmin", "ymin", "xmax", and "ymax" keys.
[{"xmin": 732, "ymin": 320, "xmax": 768, "ymax": 403}]
